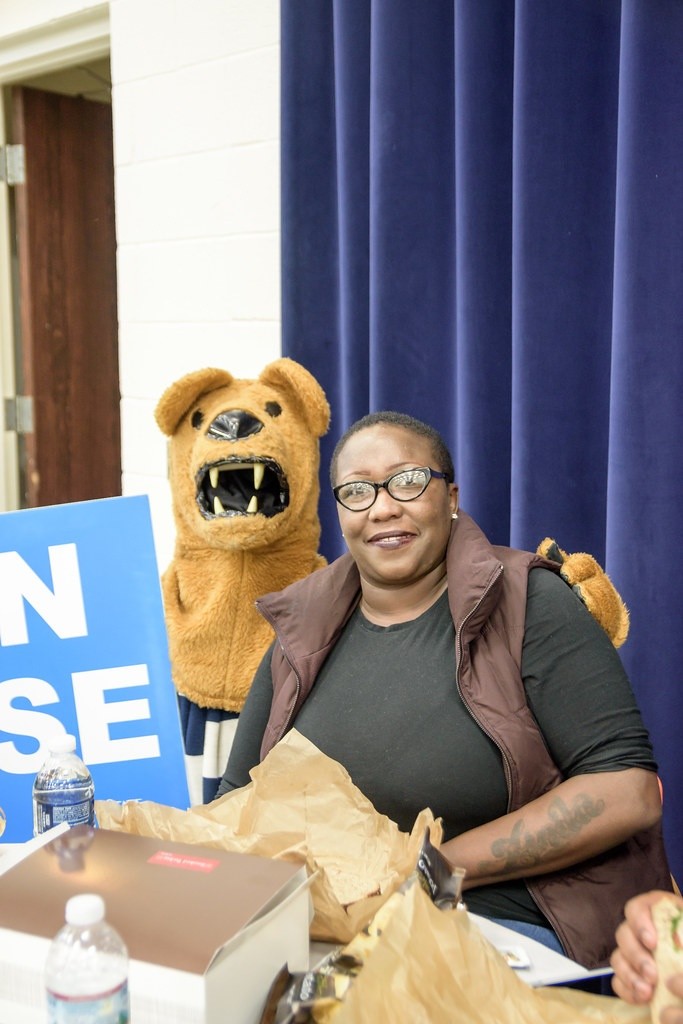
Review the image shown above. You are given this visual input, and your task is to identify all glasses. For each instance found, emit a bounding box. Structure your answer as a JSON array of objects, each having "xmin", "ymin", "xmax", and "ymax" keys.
[{"xmin": 332, "ymin": 466, "xmax": 448, "ymax": 512}]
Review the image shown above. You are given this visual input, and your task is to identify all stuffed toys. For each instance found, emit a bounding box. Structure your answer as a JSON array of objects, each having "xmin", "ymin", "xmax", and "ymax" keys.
[{"xmin": 155, "ymin": 358, "xmax": 630, "ymax": 808}]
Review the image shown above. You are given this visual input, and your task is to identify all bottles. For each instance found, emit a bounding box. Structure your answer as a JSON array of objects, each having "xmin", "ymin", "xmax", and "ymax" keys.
[
  {"xmin": 31, "ymin": 734, "xmax": 96, "ymax": 838},
  {"xmin": 40, "ymin": 893, "xmax": 130, "ymax": 1024}
]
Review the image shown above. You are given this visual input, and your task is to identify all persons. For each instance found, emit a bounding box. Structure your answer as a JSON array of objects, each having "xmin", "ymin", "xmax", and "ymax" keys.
[
  {"xmin": 609, "ymin": 890, "xmax": 683, "ymax": 1024},
  {"xmin": 210, "ymin": 411, "xmax": 675, "ymax": 996}
]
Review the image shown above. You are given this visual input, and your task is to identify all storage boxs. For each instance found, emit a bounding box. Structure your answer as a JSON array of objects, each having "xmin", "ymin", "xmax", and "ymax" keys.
[{"xmin": 0, "ymin": 822, "xmax": 320, "ymax": 1023}]
